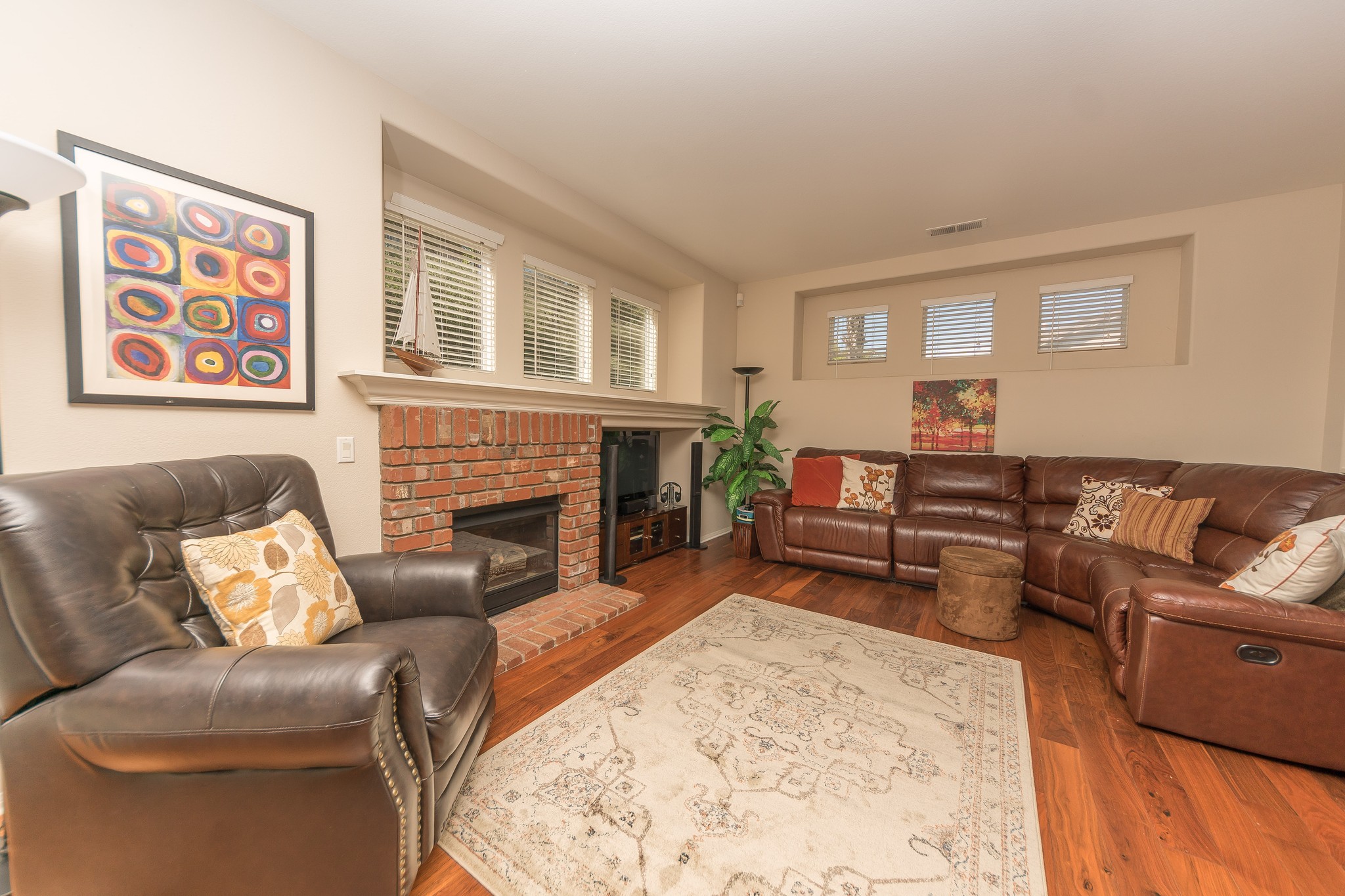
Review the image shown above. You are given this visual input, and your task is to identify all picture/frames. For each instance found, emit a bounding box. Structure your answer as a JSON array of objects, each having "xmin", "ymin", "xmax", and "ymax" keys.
[{"xmin": 57, "ymin": 130, "xmax": 315, "ymax": 411}]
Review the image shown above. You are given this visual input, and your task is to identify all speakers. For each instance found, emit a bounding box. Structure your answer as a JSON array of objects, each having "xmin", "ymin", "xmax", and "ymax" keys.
[
  {"xmin": 681, "ymin": 442, "xmax": 708, "ymax": 550},
  {"xmin": 596, "ymin": 444, "xmax": 627, "ymax": 586}
]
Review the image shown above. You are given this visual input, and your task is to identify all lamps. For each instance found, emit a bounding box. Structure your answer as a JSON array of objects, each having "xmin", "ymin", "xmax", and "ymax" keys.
[
  {"xmin": 729, "ymin": 367, "xmax": 765, "ymax": 539},
  {"xmin": 0, "ymin": 132, "xmax": 87, "ymax": 218}
]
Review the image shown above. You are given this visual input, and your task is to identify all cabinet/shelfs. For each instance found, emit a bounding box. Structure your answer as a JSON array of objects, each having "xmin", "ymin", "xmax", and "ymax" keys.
[{"xmin": 599, "ymin": 500, "xmax": 687, "ymax": 576}]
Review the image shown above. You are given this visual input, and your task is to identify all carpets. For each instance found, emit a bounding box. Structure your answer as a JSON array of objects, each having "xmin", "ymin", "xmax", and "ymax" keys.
[{"xmin": 437, "ymin": 594, "xmax": 1048, "ymax": 896}]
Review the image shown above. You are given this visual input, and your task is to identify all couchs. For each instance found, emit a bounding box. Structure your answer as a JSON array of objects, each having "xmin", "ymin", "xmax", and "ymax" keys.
[
  {"xmin": 751, "ymin": 447, "xmax": 1345, "ymax": 771},
  {"xmin": 0, "ymin": 452, "xmax": 498, "ymax": 896}
]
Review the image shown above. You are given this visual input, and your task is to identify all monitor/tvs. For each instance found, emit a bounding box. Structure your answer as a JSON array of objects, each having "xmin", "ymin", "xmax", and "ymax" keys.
[{"xmin": 597, "ymin": 430, "xmax": 661, "ymax": 517}]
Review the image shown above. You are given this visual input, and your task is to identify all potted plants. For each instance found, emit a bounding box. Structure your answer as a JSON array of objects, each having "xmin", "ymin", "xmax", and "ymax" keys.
[{"xmin": 701, "ymin": 398, "xmax": 793, "ymax": 560}]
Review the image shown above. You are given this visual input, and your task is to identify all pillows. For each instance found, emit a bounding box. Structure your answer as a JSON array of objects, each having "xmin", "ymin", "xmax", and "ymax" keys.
[
  {"xmin": 791, "ymin": 454, "xmax": 898, "ymax": 516},
  {"xmin": 1218, "ymin": 515, "xmax": 1345, "ymax": 604},
  {"xmin": 1062, "ymin": 475, "xmax": 1216, "ymax": 564},
  {"xmin": 179, "ymin": 507, "xmax": 363, "ymax": 646}
]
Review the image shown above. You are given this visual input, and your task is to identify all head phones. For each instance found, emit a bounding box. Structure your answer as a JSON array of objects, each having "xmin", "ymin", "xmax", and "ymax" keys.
[{"xmin": 660, "ymin": 482, "xmax": 681, "ymax": 503}]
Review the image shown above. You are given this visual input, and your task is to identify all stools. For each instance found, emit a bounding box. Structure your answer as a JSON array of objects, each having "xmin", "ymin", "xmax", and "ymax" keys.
[{"xmin": 936, "ymin": 546, "xmax": 1024, "ymax": 640}]
